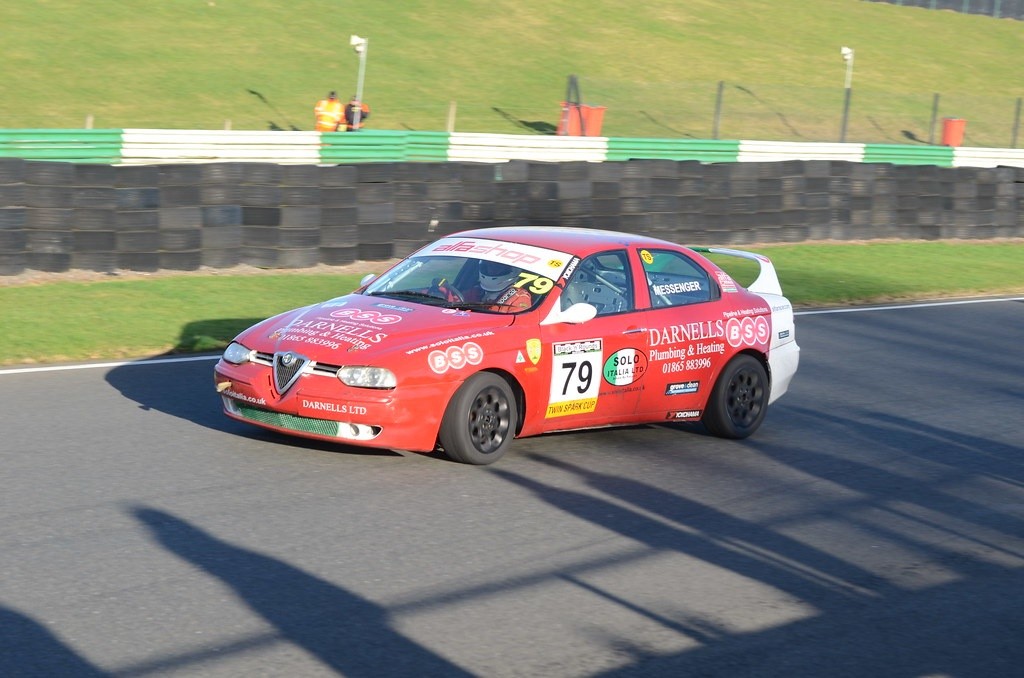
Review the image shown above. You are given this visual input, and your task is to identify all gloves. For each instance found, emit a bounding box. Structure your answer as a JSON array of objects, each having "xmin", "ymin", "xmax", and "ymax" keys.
[{"xmin": 432, "ymin": 277, "xmax": 454, "ymax": 302}]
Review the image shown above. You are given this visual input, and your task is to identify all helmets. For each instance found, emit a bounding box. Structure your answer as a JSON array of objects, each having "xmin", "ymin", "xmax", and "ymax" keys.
[{"xmin": 479, "ymin": 259, "xmax": 522, "ymax": 292}]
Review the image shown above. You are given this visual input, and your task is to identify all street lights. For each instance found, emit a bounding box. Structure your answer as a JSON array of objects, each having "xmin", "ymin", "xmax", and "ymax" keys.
[{"xmin": 351, "ymin": 35, "xmax": 369, "ymax": 130}]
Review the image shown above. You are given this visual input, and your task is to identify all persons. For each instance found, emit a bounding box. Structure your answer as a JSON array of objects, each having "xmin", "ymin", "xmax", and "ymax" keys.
[
  {"xmin": 422, "ymin": 259, "xmax": 532, "ymax": 313},
  {"xmin": 314, "ymin": 90, "xmax": 369, "ymax": 132}
]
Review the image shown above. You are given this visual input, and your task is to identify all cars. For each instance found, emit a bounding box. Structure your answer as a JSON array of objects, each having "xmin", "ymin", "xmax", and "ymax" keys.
[
  {"xmin": 839, "ymin": 48, "xmax": 855, "ymax": 144},
  {"xmin": 214, "ymin": 227, "xmax": 801, "ymax": 464}
]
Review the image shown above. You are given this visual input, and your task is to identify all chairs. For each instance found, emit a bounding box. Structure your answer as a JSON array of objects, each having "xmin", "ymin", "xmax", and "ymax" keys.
[{"xmin": 560, "ymin": 281, "xmax": 627, "ymax": 314}]
